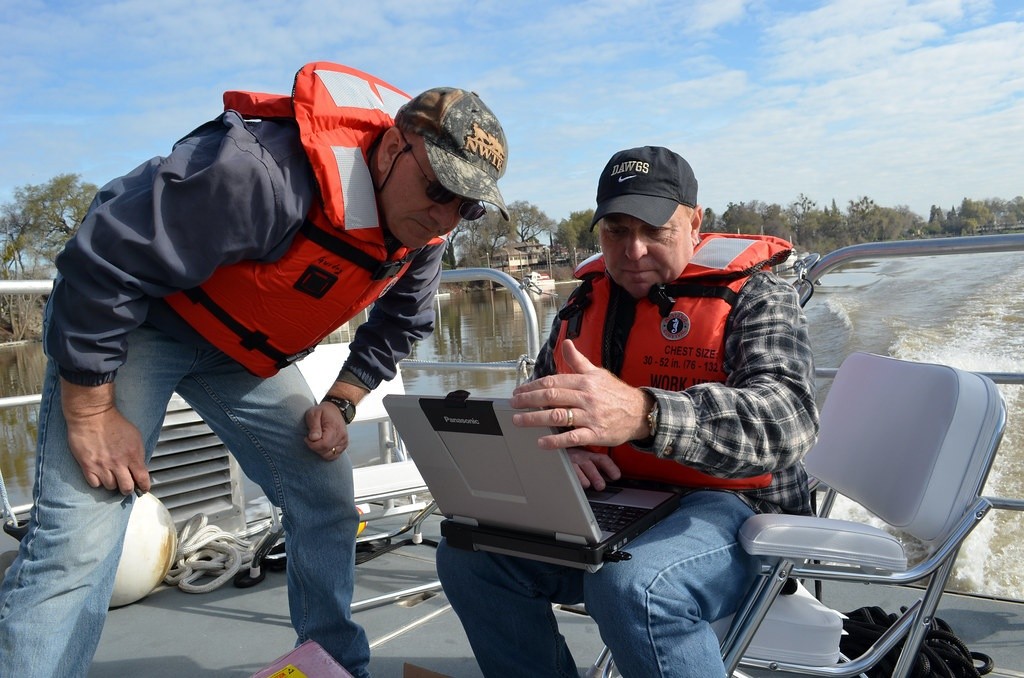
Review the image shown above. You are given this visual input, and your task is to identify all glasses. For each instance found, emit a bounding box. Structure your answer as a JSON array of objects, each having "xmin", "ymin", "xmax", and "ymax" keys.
[{"xmin": 401, "ymin": 131, "xmax": 487, "ymax": 220}]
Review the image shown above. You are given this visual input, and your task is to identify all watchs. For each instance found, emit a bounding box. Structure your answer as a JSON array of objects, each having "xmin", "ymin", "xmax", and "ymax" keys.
[
  {"xmin": 645, "ymin": 401, "xmax": 663, "ymax": 437},
  {"xmin": 321, "ymin": 392, "xmax": 357, "ymax": 425}
]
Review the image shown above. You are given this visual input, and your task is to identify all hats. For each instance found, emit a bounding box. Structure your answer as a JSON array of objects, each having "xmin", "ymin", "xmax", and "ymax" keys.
[
  {"xmin": 590, "ymin": 146, "xmax": 698, "ymax": 233},
  {"xmin": 395, "ymin": 87, "xmax": 510, "ymax": 220}
]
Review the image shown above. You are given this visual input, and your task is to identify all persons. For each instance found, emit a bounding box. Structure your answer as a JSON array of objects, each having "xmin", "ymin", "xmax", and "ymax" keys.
[
  {"xmin": 0, "ymin": 58, "xmax": 511, "ymax": 678},
  {"xmin": 433, "ymin": 145, "xmax": 823, "ymax": 678}
]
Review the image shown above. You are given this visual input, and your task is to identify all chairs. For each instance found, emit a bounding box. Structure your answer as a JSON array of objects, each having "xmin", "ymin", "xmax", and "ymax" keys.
[{"xmin": 584, "ymin": 347, "xmax": 1009, "ymax": 678}]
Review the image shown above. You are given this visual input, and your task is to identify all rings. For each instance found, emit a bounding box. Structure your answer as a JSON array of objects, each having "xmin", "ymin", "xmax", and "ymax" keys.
[
  {"xmin": 330, "ymin": 447, "xmax": 338, "ymax": 455},
  {"xmin": 565, "ymin": 407, "xmax": 574, "ymax": 428}
]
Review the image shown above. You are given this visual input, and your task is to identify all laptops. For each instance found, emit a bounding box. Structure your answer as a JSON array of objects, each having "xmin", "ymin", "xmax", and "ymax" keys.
[{"xmin": 382, "ymin": 394, "xmax": 682, "ymax": 566}]
